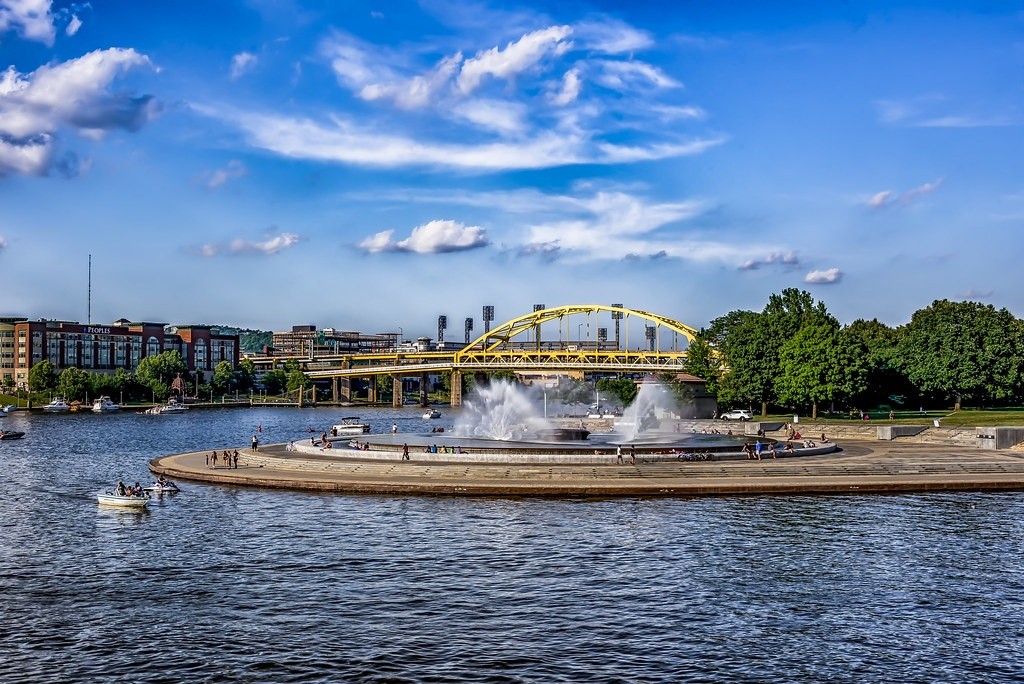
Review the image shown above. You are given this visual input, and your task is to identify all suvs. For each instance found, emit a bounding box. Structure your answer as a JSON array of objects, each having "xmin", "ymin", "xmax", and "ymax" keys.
[{"xmin": 720, "ymin": 409, "xmax": 753, "ymax": 422}]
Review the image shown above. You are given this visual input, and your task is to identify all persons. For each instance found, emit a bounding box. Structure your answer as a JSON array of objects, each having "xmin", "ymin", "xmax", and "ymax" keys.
[
  {"xmin": 157, "ymin": 474, "xmax": 166, "ymax": 488},
  {"xmin": 210, "ymin": 451, "xmax": 218, "ymax": 468},
  {"xmin": 251, "ymin": 435, "xmax": 258, "ymax": 452},
  {"xmin": 889, "ymin": 410, "xmax": 895, "ymax": 420},
  {"xmin": 865, "ymin": 414, "xmax": 869, "ymax": 421},
  {"xmin": 113, "ymin": 481, "xmax": 150, "ymax": 499},
  {"xmin": 205, "ymin": 454, "xmax": 210, "ymax": 468},
  {"xmin": 222, "ymin": 450, "xmax": 232, "ymax": 468},
  {"xmin": 286, "ymin": 402, "xmax": 864, "ymax": 466},
  {"xmin": 232, "ymin": 449, "xmax": 240, "ymax": 469},
  {"xmin": 257, "ymin": 423, "xmax": 262, "ymax": 433}
]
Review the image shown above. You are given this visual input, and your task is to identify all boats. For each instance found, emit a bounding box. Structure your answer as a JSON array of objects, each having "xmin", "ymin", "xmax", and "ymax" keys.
[
  {"xmin": 0, "ymin": 431, "xmax": 27, "ymax": 441},
  {"xmin": 101, "ymin": 396, "xmax": 120, "ymax": 410},
  {"xmin": 333, "ymin": 416, "xmax": 371, "ymax": 434},
  {"xmin": 3, "ymin": 406, "xmax": 13, "ymax": 413},
  {"xmin": 157, "ymin": 397, "xmax": 190, "ymax": 414},
  {"xmin": 422, "ymin": 408, "xmax": 442, "ymax": 419},
  {"xmin": 91, "ymin": 396, "xmax": 107, "ymax": 413},
  {"xmin": 0, "ymin": 408, "xmax": 7, "ymax": 417},
  {"xmin": 70, "ymin": 405, "xmax": 81, "ymax": 411},
  {"xmin": 43, "ymin": 400, "xmax": 70, "ymax": 414},
  {"xmin": 96, "ymin": 491, "xmax": 149, "ymax": 506},
  {"xmin": 143, "ymin": 486, "xmax": 180, "ymax": 496}
]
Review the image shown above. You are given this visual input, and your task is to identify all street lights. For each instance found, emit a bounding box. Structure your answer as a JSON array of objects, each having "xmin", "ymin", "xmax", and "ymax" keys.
[
  {"xmin": 578, "ymin": 324, "xmax": 583, "ymax": 341},
  {"xmin": 398, "ymin": 327, "xmax": 402, "ymax": 344}
]
